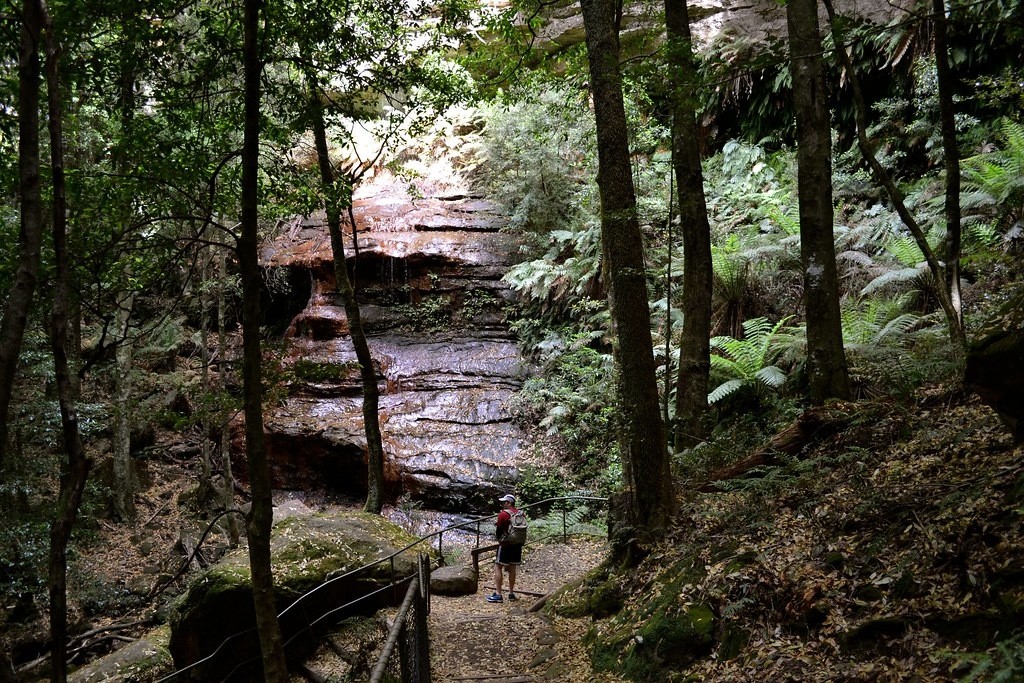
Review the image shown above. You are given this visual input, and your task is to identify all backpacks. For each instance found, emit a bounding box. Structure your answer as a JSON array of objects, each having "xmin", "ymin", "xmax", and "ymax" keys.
[{"xmin": 499, "ymin": 509, "xmax": 528, "ymax": 546}]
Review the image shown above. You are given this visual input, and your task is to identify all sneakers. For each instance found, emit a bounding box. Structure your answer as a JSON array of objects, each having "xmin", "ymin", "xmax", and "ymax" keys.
[
  {"xmin": 508, "ymin": 594, "xmax": 515, "ymax": 600},
  {"xmin": 486, "ymin": 593, "xmax": 503, "ymax": 602}
]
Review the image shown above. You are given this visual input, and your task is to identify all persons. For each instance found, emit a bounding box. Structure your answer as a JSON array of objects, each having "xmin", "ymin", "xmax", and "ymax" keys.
[{"xmin": 485, "ymin": 495, "xmax": 525, "ymax": 603}]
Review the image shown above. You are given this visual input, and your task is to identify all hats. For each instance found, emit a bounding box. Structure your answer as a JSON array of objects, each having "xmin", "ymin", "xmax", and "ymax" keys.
[{"xmin": 499, "ymin": 494, "xmax": 516, "ymax": 502}]
